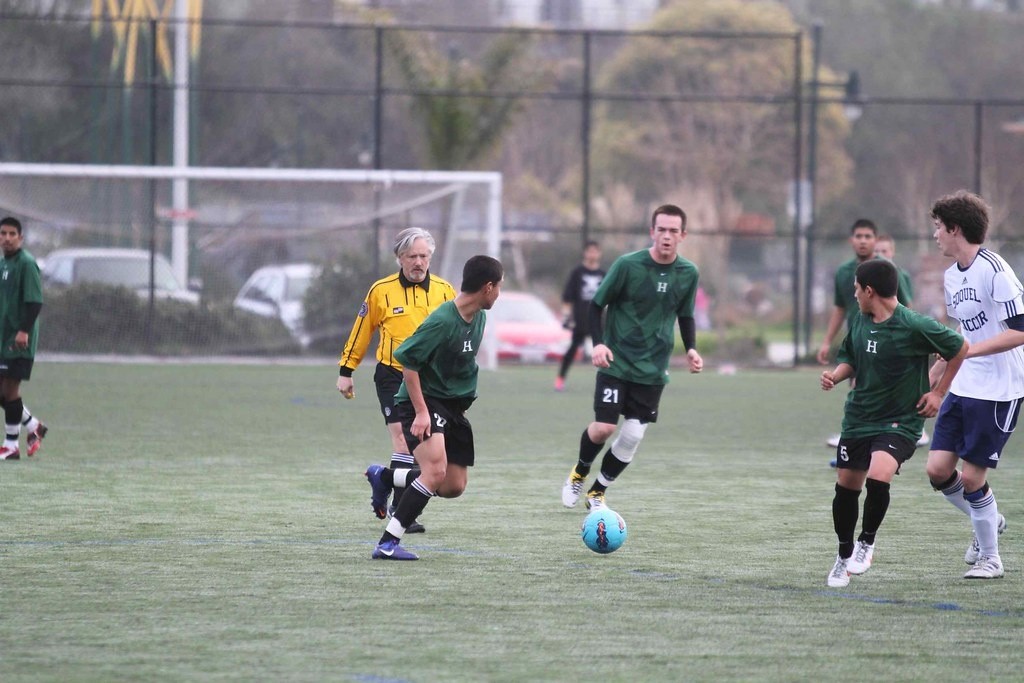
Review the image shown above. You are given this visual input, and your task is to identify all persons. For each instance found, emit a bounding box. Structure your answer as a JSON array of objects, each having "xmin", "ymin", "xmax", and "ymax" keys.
[
  {"xmin": 561, "ymin": 204, "xmax": 703, "ymax": 512},
  {"xmin": 816, "ymin": 218, "xmax": 930, "ymax": 448},
  {"xmin": 364, "ymin": 254, "xmax": 504, "ymax": 561},
  {"xmin": 552, "ymin": 239, "xmax": 608, "ymax": 392},
  {"xmin": 874, "ymin": 234, "xmax": 915, "ymax": 301},
  {"xmin": 0, "ymin": 216, "xmax": 48, "ymax": 460},
  {"xmin": 925, "ymin": 187, "xmax": 1024, "ymax": 579},
  {"xmin": 336, "ymin": 226, "xmax": 457, "ymax": 533},
  {"xmin": 820, "ymin": 259, "xmax": 970, "ymax": 588}
]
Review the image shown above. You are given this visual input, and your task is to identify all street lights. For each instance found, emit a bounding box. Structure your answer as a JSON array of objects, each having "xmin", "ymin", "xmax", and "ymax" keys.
[{"xmin": 804, "ymin": 24, "xmax": 863, "ymax": 361}]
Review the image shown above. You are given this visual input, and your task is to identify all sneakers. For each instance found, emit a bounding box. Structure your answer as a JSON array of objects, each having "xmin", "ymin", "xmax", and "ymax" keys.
[
  {"xmin": 964, "ymin": 554, "xmax": 1004, "ymax": 578},
  {"xmin": 964, "ymin": 514, "xmax": 1007, "ymax": 564},
  {"xmin": 372, "ymin": 540, "xmax": 420, "ymax": 560},
  {"xmin": 848, "ymin": 540, "xmax": 875, "ymax": 574},
  {"xmin": 0, "ymin": 446, "xmax": 21, "ymax": 460},
  {"xmin": 584, "ymin": 491, "xmax": 606, "ymax": 514},
  {"xmin": 387, "ymin": 505, "xmax": 425, "ymax": 533},
  {"xmin": 27, "ymin": 422, "xmax": 48, "ymax": 456},
  {"xmin": 365, "ymin": 464, "xmax": 392, "ymax": 518},
  {"xmin": 826, "ymin": 555, "xmax": 851, "ymax": 588},
  {"xmin": 562, "ymin": 464, "xmax": 589, "ymax": 508}
]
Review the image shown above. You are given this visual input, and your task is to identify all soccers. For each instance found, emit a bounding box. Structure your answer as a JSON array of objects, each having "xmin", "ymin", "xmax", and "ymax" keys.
[{"xmin": 581, "ymin": 509, "xmax": 627, "ymax": 554}]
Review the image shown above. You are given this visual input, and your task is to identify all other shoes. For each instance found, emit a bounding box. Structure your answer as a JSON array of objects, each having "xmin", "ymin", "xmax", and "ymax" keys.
[
  {"xmin": 553, "ymin": 374, "xmax": 565, "ymax": 391},
  {"xmin": 830, "ymin": 460, "xmax": 838, "ymax": 466},
  {"xmin": 917, "ymin": 429, "xmax": 931, "ymax": 447},
  {"xmin": 827, "ymin": 435, "xmax": 840, "ymax": 447}
]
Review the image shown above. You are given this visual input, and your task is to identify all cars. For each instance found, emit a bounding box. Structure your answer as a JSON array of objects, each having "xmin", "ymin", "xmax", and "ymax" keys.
[
  {"xmin": 233, "ymin": 263, "xmax": 345, "ymax": 348},
  {"xmin": 475, "ymin": 291, "xmax": 592, "ymax": 369},
  {"xmin": 37, "ymin": 250, "xmax": 198, "ymax": 307}
]
